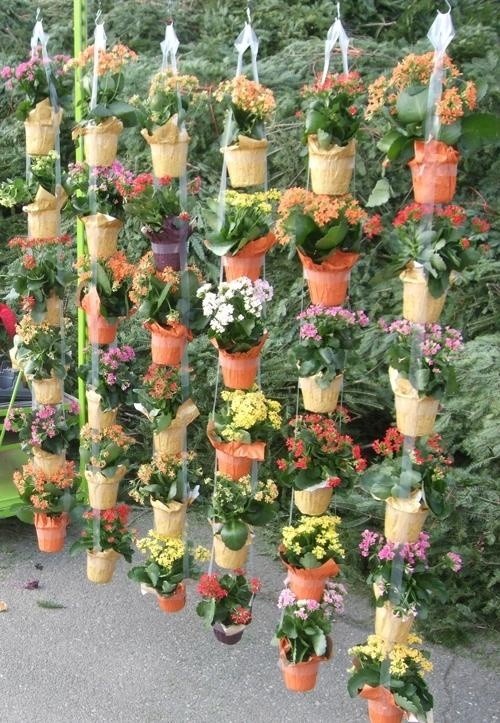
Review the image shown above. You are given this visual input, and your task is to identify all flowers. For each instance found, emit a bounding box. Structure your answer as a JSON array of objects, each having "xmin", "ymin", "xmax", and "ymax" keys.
[{"xmin": 1, "ymin": 38, "xmax": 475, "ymax": 722}]
